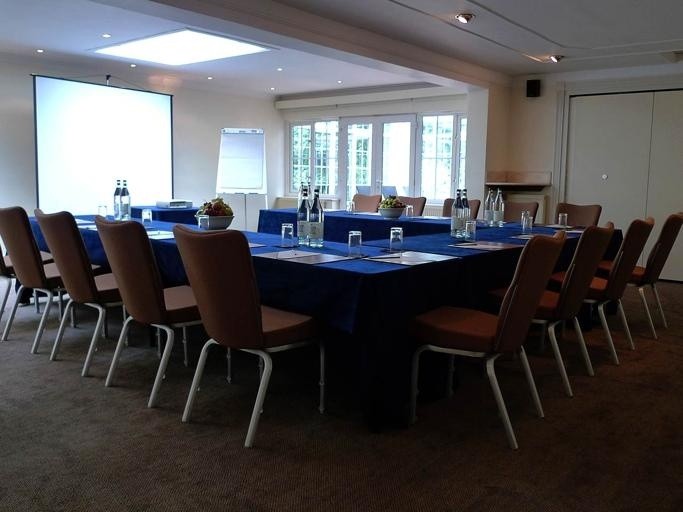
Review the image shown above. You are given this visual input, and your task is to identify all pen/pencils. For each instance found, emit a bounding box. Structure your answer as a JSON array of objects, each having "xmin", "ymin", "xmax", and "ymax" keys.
[
  {"xmin": 454, "ymin": 244, "xmax": 478, "ymax": 246},
  {"xmin": 370, "ymin": 256, "xmax": 400, "ymax": 259}
]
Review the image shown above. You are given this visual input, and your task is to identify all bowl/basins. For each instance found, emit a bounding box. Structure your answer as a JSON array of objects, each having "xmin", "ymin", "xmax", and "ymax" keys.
[
  {"xmin": 194, "ymin": 215, "xmax": 234, "ymax": 230},
  {"xmin": 376, "ymin": 207, "xmax": 403, "ymax": 219}
]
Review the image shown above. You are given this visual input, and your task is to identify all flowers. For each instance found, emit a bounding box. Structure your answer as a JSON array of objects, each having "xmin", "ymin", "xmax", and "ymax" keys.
[{"xmin": 377, "ymin": 195, "xmax": 408, "ymax": 207}]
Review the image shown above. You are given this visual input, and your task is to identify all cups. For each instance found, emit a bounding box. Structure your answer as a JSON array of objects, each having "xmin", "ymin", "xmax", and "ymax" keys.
[
  {"xmin": 197, "ymin": 215, "xmax": 209, "ymax": 231},
  {"xmin": 465, "ymin": 220, "xmax": 476, "ymax": 241},
  {"xmin": 519, "ymin": 210, "xmax": 533, "ymax": 234},
  {"xmin": 347, "ymin": 230, "xmax": 361, "ymax": 257},
  {"xmin": 405, "ymin": 205, "xmax": 413, "ymax": 217},
  {"xmin": 347, "ymin": 201, "xmax": 355, "ymax": 215},
  {"xmin": 389, "ymin": 227, "xmax": 403, "ymax": 252},
  {"xmin": 280, "ymin": 223, "xmax": 293, "ymax": 246},
  {"xmin": 558, "ymin": 212, "xmax": 567, "ymax": 227},
  {"xmin": 97, "ymin": 206, "xmax": 107, "ymax": 219},
  {"xmin": 140, "ymin": 208, "xmax": 152, "ymax": 227}
]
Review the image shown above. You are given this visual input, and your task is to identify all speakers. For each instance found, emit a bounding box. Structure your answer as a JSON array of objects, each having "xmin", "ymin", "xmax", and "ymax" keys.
[{"xmin": 526, "ymin": 79, "xmax": 541, "ymax": 97}]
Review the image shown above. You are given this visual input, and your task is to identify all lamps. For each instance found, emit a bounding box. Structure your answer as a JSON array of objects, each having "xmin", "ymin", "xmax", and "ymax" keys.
[{"xmin": 550, "ymin": 55, "xmax": 565, "ymax": 64}]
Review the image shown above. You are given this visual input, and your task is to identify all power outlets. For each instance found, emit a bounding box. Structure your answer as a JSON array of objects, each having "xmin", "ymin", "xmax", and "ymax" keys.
[{"xmin": 454, "ymin": 11, "xmax": 479, "ymax": 26}]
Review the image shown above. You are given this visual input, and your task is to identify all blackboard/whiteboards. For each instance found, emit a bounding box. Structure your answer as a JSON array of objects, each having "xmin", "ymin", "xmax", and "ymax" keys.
[{"xmin": 216, "ymin": 128, "xmax": 267, "ymax": 194}]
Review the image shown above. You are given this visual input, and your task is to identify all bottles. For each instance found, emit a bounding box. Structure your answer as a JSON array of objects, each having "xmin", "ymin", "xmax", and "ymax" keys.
[
  {"xmin": 113, "ymin": 179, "xmax": 131, "ymax": 222},
  {"xmin": 296, "ymin": 189, "xmax": 325, "ymax": 248},
  {"xmin": 450, "ymin": 188, "xmax": 469, "ymax": 237},
  {"xmin": 483, "ymin": 185, "xmax": 504, "ymax": 227}
]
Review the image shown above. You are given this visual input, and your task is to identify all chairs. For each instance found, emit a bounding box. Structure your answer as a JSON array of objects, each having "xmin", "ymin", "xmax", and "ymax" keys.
[
  {"xmin": 409, "ymin": 228, "xmax": 568, "ymax": 447},
  {"xmin": 502, "ymin": 201, "xmax": 539, "ymax": 222},
  {"xmin": 396, "ymin": 197, "xmax": 427, "ymax": 215},
  {"xmin": 3, "ymin": 209, "xmax": 682, "ymax": 426},
  {"xmin": 553, "ymin": 202, "xmax": 602, "ymax": 225},
  {"xmin": 170, "ymin": 224, "xmax": 323, "ymax": 447},
  {"xmin": 353, "ymin": 193, "xmax": 382, "ymax": 212},
  {"xmin": 441, "ymin": 198, "xmax": 480, "ymax": 218}
]
